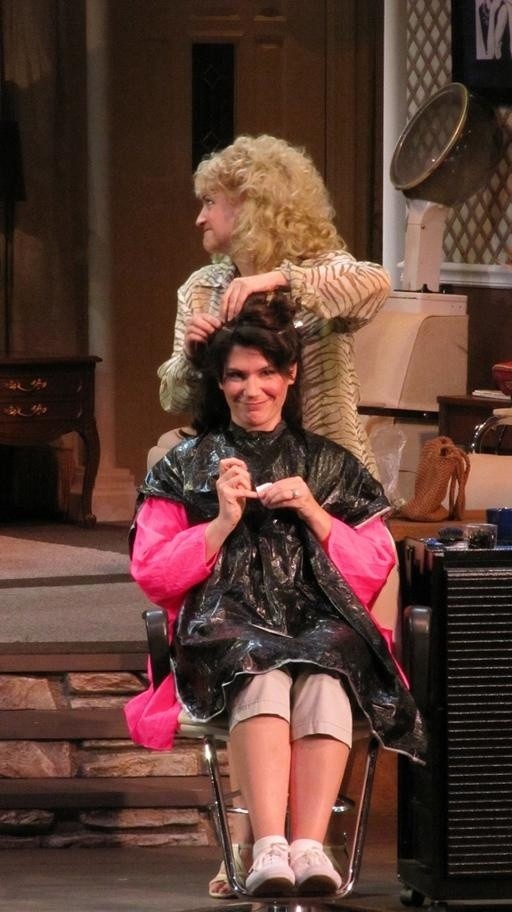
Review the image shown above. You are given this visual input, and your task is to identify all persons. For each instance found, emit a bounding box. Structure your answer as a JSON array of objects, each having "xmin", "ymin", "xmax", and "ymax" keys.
[
  {"xmin": 158, "ymin": 132, "xmax": 390, "ymax": 904},
  {"xmin": 127, "ymin": 285, "xmax": 426, "ymax": 901}
]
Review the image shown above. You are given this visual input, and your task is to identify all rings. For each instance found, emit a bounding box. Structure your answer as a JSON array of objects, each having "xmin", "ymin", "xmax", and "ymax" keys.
[{"xmin": 290, "ymin": 490, "xmax": 299, "ymax": 500}]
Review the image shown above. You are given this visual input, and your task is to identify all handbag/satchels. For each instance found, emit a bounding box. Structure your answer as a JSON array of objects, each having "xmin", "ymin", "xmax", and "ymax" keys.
[{"xmin": 391, "ymin": 436, "xmax": 472, "ymax": 522}]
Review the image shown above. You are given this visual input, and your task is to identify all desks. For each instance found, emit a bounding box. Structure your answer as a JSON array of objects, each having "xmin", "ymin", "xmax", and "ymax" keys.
[{"xmin": 0, "ymin": 356, "xmax": 102, "ymax": 528}]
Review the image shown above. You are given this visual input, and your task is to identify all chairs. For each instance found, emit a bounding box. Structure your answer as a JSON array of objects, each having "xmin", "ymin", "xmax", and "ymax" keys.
[{"xmin": 143, "ymin": 427, "xmax": 434, "ymax": 912}]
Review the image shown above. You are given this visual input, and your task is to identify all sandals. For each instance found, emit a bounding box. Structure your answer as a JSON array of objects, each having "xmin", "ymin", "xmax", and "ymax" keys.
[{"xmin": 207, "ymin": 843, "xmax": 246, "ymax": 899}]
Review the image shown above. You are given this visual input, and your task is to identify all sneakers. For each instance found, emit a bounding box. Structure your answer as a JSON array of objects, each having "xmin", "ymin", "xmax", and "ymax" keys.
[
  {"xmin": 290, "ymin": 849, "xmax": 342, "ymax": 895},
  {"xmin": 243, "ymin": 849, "xmax": 296, "ymax": 896}
]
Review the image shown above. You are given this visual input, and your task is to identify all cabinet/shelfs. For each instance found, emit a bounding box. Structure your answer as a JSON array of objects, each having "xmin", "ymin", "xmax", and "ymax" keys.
[{"xmin": 398, "ymin": 537, "xmax": 512, "ymax": 911}]
